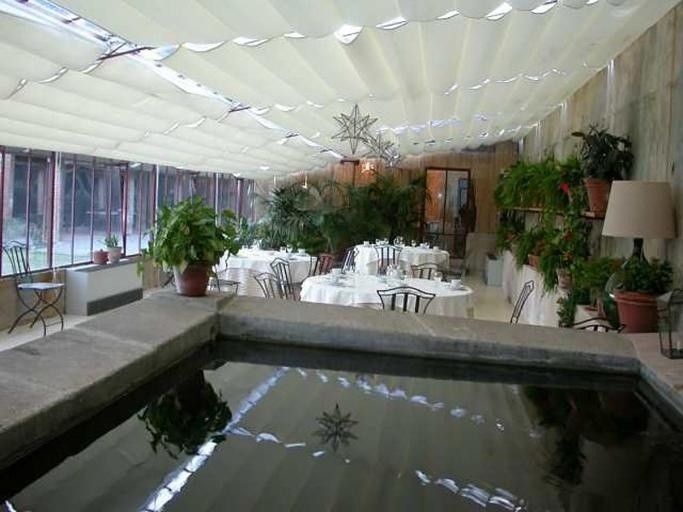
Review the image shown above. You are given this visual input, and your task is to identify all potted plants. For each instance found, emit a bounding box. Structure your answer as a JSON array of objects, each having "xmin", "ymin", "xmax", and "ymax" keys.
[
  {"xmin": 135, "ymin": 194, "xmax": 243, "ymax": 298},
  {"xmin": 137, "ymin": 368, "xmax": 235, "ymax": 461},
  {"xmin": 97, "ymin": 233, "xmax": 122, "ymax": 266}
]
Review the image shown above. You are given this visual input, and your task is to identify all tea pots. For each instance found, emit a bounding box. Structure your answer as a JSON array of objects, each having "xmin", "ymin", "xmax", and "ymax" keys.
[{"xmin": 392, "ymin": 235, "xmax": 405, "ymax": 248}]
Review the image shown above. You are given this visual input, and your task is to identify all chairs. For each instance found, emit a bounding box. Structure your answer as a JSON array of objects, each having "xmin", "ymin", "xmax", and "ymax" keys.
[{"xmin": 1, "ymin": 239, "xmax": 67, "ymax": 339}]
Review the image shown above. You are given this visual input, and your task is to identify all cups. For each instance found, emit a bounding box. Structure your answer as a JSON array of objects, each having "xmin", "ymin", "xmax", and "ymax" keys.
[
  {"xmin": 279, "ymin": 244, "xmax": 306, "ymax": 260},
  {"xmin": 240, "ymin": 244, "xmax": 258, "ymax": 257},
  {"xmin": 431, "ymin": 271, "xmax": 462, "ymax": 292},
  {"xmin": 360, "ymin": 234, "xmax": 389, "ymax": 248},
  {"xmin": 328, "ymin": 266, "xmax": 357, "ymax": 288},
  {"xmin": 410, "ymin": 238, "xmax": 441, "ymax": 253},
  {"xmin": 383, "ymin": 261, "xmax": 413, "ymax": 288}
]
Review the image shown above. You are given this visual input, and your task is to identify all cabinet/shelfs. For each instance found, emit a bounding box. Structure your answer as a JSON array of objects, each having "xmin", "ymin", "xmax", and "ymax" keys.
[{"xmin": 65, "ymin": 257, "xmax": 145, "ymax": 318}]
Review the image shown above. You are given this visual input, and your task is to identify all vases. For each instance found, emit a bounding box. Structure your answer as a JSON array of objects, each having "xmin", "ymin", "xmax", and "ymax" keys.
[{"xmin": 92, "ymin": 250, "xmax": 108, "ymax": 265}]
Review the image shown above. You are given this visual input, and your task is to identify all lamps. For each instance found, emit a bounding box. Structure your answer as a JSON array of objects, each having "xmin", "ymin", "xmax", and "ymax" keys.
[{"xmin": 651, "ymin": 287, "xmax": 683, "ymax": 359}]
